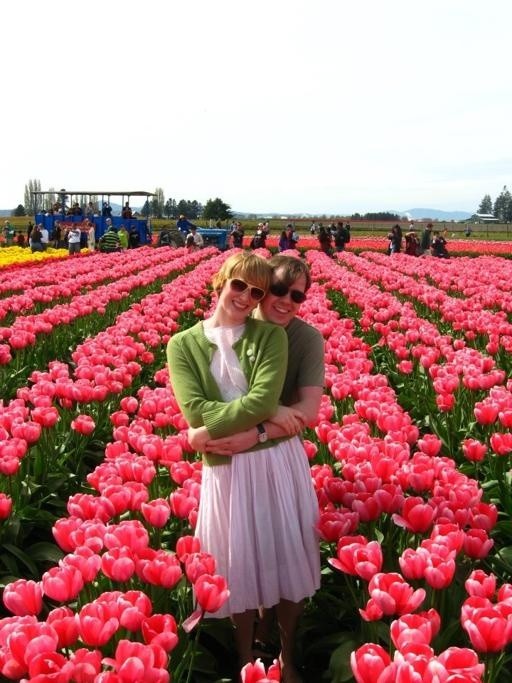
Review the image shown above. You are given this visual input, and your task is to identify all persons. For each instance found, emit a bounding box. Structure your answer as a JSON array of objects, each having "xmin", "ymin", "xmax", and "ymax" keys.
[
  {"xmin": 386, "ymin": 220, "xmax": 472, "ymax": 259},
  {"xmin": 1, "ymin": 198, "xmax": 140, "ymax": 254},
  {"xmin": 205, "ymin": 254, "xmax": 326, "ymax": 454},
  {"xmin": 167, "ymin": 215, "xmax": 351, "ymax": 253},
  {"xmin": 165, "ymin": 251, "xmax": 305, "ymax": 682}
]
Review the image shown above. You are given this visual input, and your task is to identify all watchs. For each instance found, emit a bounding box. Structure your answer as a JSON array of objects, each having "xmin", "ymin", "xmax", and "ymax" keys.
[{"xmin": 257, "ymin": 421, "xmax": 270, "ymax": 443}]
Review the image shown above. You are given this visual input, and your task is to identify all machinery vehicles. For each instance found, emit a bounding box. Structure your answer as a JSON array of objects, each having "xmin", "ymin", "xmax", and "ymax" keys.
[{"xmin": 159, "ymin": 223, "xmax": 230, "ymax": 252}]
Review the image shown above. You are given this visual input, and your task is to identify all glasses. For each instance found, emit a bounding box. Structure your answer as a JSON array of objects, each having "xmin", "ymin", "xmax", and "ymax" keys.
[
  {"xmin": 270, "ymin": 284, "xmax": 307, "ymax": 303},
  {"xmin": 227, "ymin": 277, "xmax": 266, "ymax": 302}
]
